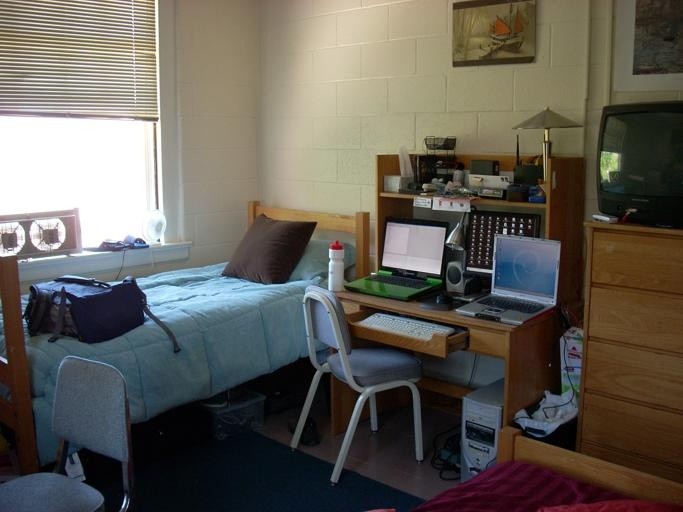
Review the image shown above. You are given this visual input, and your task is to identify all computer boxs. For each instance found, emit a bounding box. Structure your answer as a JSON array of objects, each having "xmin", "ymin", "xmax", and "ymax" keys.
[{"xmin": 460, "ymin": 378, "xmax": 503, "ymax": 484}]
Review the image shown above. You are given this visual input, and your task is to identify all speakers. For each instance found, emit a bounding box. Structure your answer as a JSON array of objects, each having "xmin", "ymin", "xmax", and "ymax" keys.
[{"xmin": 444, "ymin": 261, "xmax": 465, "ymax": 293}]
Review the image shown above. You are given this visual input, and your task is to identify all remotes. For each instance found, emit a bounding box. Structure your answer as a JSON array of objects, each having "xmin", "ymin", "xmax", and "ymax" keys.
[{"xmin": 591, "ymin": 213, "xmax": 618, "ymax": 224}]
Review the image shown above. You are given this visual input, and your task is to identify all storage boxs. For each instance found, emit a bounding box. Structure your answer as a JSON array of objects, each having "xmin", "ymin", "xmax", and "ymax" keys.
[{"xmin": 195, "ymin": 385, "xmax": 267, "ymax": 441}]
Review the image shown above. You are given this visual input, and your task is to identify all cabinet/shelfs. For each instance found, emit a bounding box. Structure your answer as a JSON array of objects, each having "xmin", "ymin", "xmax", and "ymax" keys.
[
  {"xmin": 329, "ymin": 154, "xmax": 585, "ymax": 466},
  {"xmin": 575, "ymin": 219, "xmax": 683, "ymax": 486}
]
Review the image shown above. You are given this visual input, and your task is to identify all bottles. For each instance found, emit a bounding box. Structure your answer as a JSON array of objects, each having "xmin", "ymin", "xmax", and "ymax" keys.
[{"xmin": 328, "ymin": 239, "xmax": 345, "ymax": 291}]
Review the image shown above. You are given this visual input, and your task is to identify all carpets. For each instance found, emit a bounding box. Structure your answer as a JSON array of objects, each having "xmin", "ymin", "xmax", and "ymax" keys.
[{"xmin": 83, "ymin": 406, "xmax": 424, "ymax": 512}]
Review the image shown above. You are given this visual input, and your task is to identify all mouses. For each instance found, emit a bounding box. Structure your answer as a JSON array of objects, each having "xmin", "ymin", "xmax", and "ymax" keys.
[{"xmin": 433, "ymin": 292, "xmax": 452, "ymax": 304}]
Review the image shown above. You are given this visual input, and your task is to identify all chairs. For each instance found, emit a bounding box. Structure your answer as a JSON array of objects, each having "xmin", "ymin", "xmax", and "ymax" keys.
[
  {"xmin": 0, "ymin": 356, "xmax": 135, "ymax": 512},
  {"xmin": 291, "ymin": 286, "xmax": 428, "ymax": 486}
]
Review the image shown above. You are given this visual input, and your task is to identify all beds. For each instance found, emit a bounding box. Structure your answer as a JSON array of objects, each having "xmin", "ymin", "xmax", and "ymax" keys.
[
  {"xmin": 411, "ymin": 426, "xmax": 683, "ymax": 511},
  {"xmin": 0, "ymin": 200, "xmax": 370, "ymax": 480}
]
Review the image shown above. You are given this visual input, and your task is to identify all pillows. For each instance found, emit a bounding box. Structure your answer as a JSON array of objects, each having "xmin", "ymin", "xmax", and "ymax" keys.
[
  {"xmin": 287, "ymin": 239, "xmax": 356, "ymax": 281},
  {"xmin": 220, "ymin": 212, "xmax": 317, "ymax": 284}
]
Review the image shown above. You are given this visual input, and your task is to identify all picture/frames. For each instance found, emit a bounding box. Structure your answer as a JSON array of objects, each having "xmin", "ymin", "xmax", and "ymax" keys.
[
  {"xmin": 453, "ymin": 0, "xmax": 536, "ymax": 67},
  {"xmin": 614, "ymin": 0, "xmax": 683, "ymax": 91}
]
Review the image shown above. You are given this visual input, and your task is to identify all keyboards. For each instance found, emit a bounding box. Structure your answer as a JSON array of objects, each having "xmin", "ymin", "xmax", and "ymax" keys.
[{"xmin": 359, "ymin": 313, "xmax": 455, "ymax": 342}]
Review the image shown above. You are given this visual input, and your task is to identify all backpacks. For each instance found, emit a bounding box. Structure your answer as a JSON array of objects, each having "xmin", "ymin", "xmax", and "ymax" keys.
[{"xmin": 23, "ymin": 274, "xmax": 146, "ymax": 344}]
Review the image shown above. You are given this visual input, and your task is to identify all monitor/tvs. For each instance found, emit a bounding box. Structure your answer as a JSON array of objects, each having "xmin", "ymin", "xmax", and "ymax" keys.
[{"xmin": 595, "ymin": 99, "xmax": 682, "ymax": 232}]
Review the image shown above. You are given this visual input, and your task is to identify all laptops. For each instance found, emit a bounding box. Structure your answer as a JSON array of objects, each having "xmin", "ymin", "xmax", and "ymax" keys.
[
  {"xmin": 343, "ymin": 216, "xmax": 449, "ymax": 301},
  {"xmin": 454, "ymin": 233, "xmax": 561, "ymax": 325}
]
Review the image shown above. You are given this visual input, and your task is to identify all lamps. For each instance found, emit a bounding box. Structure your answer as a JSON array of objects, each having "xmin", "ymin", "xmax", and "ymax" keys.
[{"xmin": 512, "ymin": 105, "xmax": 583, "ymax": 184}]
